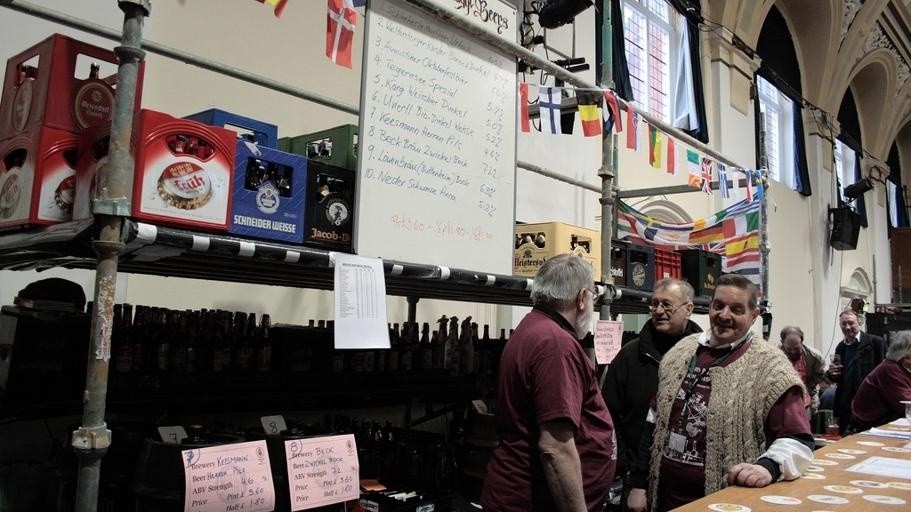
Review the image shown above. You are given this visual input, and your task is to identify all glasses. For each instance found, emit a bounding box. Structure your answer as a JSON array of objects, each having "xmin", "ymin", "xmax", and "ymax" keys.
[{"xmin": 650, "ymin": 302, "xmax": 687, "ymax": 311}]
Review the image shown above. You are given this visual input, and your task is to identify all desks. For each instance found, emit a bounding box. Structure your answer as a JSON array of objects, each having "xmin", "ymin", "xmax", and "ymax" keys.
[{"xmin": 667, "ymin": 414, "xmax": 911, "ymax": 512}]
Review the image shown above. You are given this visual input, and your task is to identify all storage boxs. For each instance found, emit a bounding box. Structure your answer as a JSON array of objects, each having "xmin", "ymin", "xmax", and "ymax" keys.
[
  {"xmin": 276, "ymin": 119, "xmax": 359, "ymax": 250},
  {"xmin": 175, "ymin": 100, "xmax": 305, "ymax": 246},
  {"xmin": 0, "ymin": 37, "xmax": 145, "ymax": 231},
  {"xmin": 513, "ymin": 220, "xmax": 602, "ymax": 280},
  {"xmin": 130, "ymin": 102, "xmax": 235, "ymax": 236},
  {"xmin": 610, "ymin": 229, "xmax": 721, "ymax": 295}
]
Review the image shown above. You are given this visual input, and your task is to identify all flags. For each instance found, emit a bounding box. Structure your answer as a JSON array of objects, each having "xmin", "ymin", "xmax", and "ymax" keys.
[
  {"xmin": 520, "ymin": 82, "xmax": 767, "ymax": 204},
  {"xmin": 325, "ymin": 1, "xmax": 359, "ymax": 69},
  {"xmin": 257, "ymin": 0, "xmax": 289, "ymax": 17},
  {"xmin": 618, "ymin": 196, "xmax": 764, "ymax": 300}
]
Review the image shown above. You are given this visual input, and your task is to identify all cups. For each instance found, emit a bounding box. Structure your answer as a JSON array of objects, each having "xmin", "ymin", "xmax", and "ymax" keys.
[{"xmin": 829, "ymin": 354, "xmax": 843, "ymax": 375}]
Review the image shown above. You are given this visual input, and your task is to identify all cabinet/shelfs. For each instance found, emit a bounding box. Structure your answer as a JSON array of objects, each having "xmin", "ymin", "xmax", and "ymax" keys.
[{"xmin": 0, "ymin": 0, "xmax": 770, "ymax": 512}]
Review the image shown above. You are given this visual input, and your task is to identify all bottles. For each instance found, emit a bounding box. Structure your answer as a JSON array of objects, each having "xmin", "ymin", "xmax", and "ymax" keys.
[
  {"xmin": 310, "ymin": 316, "xmax": 508, "ymax": 373},
  {"xmin": 86, "ymin": 62, "xmax": 104, "ymax": 81},
  {"xmin": 113, "ymin": 304, "xmax": 271, "ymax": 377},
  {"xmin": 172, "ymin": 135, "xmax": 189, "ymax": 155}
]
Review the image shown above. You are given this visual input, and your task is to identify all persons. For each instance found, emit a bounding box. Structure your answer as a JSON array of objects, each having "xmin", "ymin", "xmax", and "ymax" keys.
[
  {"xmin": 602, "ymin": 275, "xmax": 706, "ymax": 511},
  {"xmin": 844, "ymin": 330, "xmax": 911, "ymax": 436},
  {"xmin": 773, "ymin": 325, "xmax": 828, "ymax": 425},
  {"xmin": 481, "ymin": 254, "xmax": 621, "ymax": 512},
  {"xmin": 829, "ymin": 308, "xmax": 888, "ymax": 435},
  {"xmin": 623, "ymin": 272, "xmax": 817, "ymax": 512}
]
